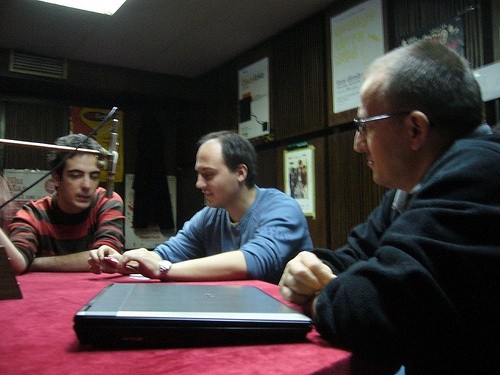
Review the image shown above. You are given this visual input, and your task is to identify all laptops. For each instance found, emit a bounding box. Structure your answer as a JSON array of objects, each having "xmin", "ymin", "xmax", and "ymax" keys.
[{"xmin": 73, "ymin": 283, "xmax": 314, "ymax": 344}]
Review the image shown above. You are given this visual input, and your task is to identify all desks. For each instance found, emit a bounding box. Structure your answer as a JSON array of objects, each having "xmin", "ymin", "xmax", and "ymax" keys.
[{"xmin": 0, "ymin": 271, "xmax": 353, "ymax": 374}]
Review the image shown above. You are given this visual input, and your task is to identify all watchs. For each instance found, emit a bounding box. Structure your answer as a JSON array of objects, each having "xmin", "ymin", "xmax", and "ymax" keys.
[{"xmin": 159, "ymin": 259, "xmax": 172, "ymax": 281}]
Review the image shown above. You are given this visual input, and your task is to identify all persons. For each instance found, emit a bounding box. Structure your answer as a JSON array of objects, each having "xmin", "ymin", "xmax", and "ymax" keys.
[
  {"xmin": 88, "ymin": 130, "xmax": 315, "ymax": 285},
  {"xmin": 279, "ymin": 39, "xmax": 500, "ymax": 375},
  {"xmin": 0, "ymin": 133, "xmax": 125, "ymax": 275}
]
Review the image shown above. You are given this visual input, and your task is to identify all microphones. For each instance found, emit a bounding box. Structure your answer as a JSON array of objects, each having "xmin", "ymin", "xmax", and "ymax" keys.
[{"xmin": 107, "ymin": 109, "xmax": 118, "ymax": 199}]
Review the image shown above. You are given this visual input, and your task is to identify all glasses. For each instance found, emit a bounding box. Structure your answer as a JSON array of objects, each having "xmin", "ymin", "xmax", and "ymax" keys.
[{"xmin": 353, "ymin": 111, "xmax": 408, "ymax": 133}]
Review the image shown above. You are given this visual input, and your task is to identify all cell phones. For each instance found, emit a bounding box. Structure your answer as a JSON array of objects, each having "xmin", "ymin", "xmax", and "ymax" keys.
[{"xmin": 103, "ymin": 257, "xmax": 136, "ymax": 274}]
